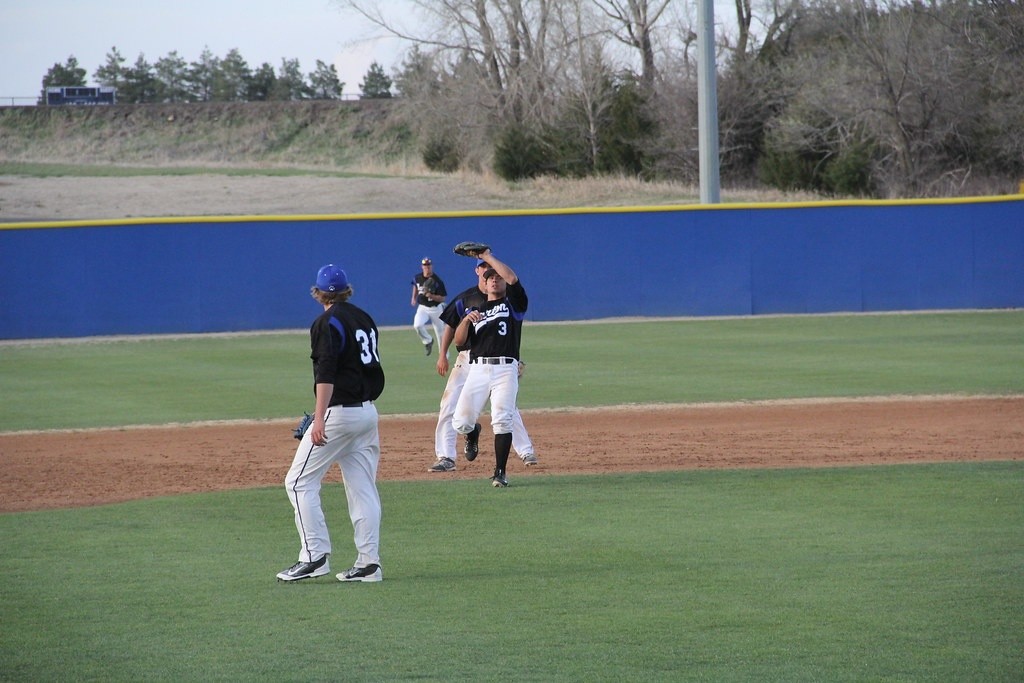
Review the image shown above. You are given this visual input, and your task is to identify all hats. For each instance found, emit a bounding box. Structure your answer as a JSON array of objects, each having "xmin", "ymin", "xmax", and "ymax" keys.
[
  {"xmin": 483, "ymin": 268, "xmax": 497, "ymax": 280},
  {"xmin": 310, "ymin": 264, "xmax": 349, "ymax": 292},
  {"xmin": 421, "ymin": 256, "xmax": 432, "ymax": 264},
  {"xmin": 477, "ymin": 253, "xmax": 500, "ymax": 266}
]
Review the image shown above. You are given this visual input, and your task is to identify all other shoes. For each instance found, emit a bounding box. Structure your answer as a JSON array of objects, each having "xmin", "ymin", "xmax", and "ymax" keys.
[{"xmin": 425, "ymin": 338, "xmax": 434, "ymax": 356}]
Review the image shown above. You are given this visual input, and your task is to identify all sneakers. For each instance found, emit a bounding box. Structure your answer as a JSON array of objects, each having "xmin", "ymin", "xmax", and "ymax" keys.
[
  {"xmin": 463, "ymin": 423, "xmax": 482, "ymax": 461},
  {"xmin": 523, "ymin": 456, "xmax": 537, "ymax": 466},
  {"xmin": 276, "ymin": 555, "xmax": 330, "ymax": 581},
  {"xmin": 428, "ymin": 456, "xmax": 457, "ymax": 472},
  {"xmin": 336, "ymin": 564, "xmax": 382, "ymax": 582},
  {"xmin": 490, "ymin": 468, "xmax": 508, "ymax": 488}
]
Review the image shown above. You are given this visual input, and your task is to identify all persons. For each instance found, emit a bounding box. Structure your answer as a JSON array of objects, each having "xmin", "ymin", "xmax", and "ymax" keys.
[
  {"xmin": 275, "ymin": 264, "xmax": 385, "ymax": 584},
  {"xmin": 410, "ymin": 257, "xmax": 450, "ymax": 360},
  {"xmin": 427, "ymin": 254, "xmax": 537, "ymax": 471},
  {"xmin": 452, "ymin": 241, "xmax": 528, "ymax": 487}
]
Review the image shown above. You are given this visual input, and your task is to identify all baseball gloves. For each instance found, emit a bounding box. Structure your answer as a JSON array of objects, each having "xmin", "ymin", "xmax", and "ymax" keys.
[
  {"xmin": 453, "ymin": 240, "xmax": 492, "ymax": 260},
  {"xmin": 424, "ymin": 277, "xmax": 437, "ymax": 294},
  {"xmin": 292, "ymin": 411, "xmax": 319, "ymax": 442}
]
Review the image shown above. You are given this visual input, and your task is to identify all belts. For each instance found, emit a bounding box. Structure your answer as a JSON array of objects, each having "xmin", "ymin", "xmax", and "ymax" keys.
[
  {"xmin": 343, "ymin": 400, "xmax": 372, "ymax": 407},
  {"xmin": 475, "ymin": 359, "xmax": 513, "ymax": 364}
]
